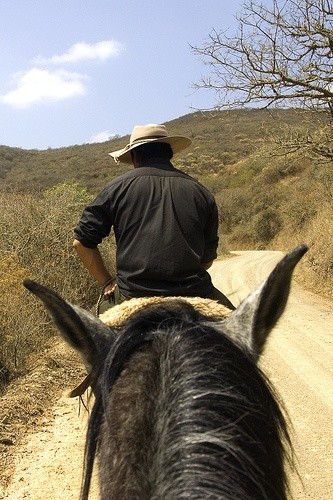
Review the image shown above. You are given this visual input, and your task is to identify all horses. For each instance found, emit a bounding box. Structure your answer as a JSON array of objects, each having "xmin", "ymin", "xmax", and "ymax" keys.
[{"xmin": 21, "ymin": 244, "xmax": 308, "ymax": 500}]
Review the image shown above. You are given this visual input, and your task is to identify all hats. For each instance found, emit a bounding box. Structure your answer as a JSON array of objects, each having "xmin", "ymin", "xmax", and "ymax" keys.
[{"xmin": 106, "ymin": 124, "xmax": 194, "ymax": 163}]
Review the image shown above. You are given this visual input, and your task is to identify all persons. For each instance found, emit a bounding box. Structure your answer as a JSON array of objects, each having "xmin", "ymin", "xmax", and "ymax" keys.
[{"xmin": 74, "ymin": 124, "xmax": 240, "ymax": 318}]
